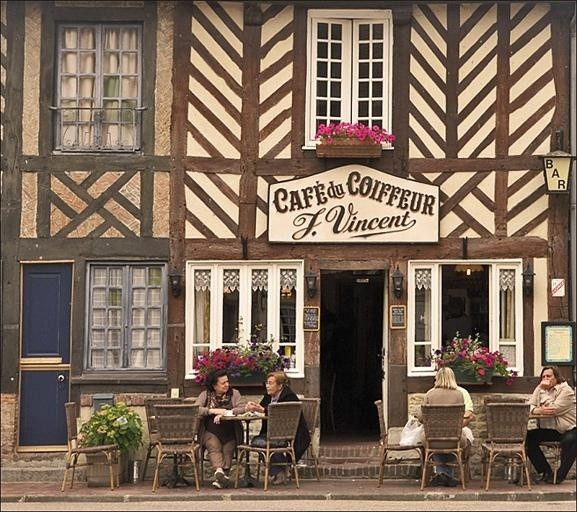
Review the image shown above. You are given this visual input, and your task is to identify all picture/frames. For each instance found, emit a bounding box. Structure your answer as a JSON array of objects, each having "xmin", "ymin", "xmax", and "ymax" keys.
[
  {"xmin": 389, "ymin": 304, "xmax": 407, "ymax": 331},
  {"xmin": 303, "ymin": 305, "xmax": 321, "ymax": 332},
  {"xmin": 540, "ymin": 319, "xmax": 577, "ymax": 368}
]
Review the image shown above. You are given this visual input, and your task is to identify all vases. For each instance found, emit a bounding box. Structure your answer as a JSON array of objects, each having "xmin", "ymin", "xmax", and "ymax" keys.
[
  {"xmin": 228, "ymin": 371, "xmax": 263, "ymax": 389},
  {"xmin": 315, "ymin": 138, "xmax": 383, "ymax": 159},
  {"xmin": 447, "ymin": 363, "xmax": 494, "ymax": 385}
]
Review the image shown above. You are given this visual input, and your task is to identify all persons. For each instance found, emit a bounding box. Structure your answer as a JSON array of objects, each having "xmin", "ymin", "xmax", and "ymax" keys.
[
  {"xmin": 245, "ymin": 371, "xmax": 312, "ymax": 485},
  {"xmin": 193, "ymin": 369, "xmax": 244, "ymax": 488},
  {"xmin": 418, "ymin": 368, "xmax": 464, "ymax": 486},
  {"xmin": 424, "ymin": 385, "xmax": 475, "ymax": 483},
  {"xmin": 525, "ymin": 365, "xmax": 577, "ymax": 484}
]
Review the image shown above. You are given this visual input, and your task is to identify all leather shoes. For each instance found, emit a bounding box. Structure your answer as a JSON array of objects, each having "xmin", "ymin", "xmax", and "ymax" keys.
[{"xmin": 536, "ymin": 470, "xmax": 563, "ymax": 484}]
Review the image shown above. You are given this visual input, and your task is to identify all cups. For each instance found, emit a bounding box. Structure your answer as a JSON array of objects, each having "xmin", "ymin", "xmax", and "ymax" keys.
[{"xmin": 224, "ymin": 410, "xmax": 234, "ymax": 415}]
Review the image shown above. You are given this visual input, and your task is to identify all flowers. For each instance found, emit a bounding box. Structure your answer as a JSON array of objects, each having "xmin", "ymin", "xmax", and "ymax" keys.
[
  {"xmin": 190, "ymin": 311, "xmax": 292, "ymax": 389},
  {"xmin": 312, "ymin": 120, "xmax": 397, "ymax": 145},
  {"xmin": 76, "ymin": 399, "xmax": 145, "ymax": 459},
  {"xmin": 417, "ymin": 326, "xmax": 519, "ymax": 387}
]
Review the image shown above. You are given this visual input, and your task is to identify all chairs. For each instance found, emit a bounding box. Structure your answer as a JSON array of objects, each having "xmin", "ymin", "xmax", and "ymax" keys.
[
  {"xmin": 419, "ymin": 401, "xmax": 468, "ymax": 491},
  {"xmin": 479, "ymin": 394, "xmax": 531, "ymax": 489},
  {"xmin": 60, "ymin": 401, "xmax": 120, "ymax": 493},
  {"xmin": 234, "ymin": 401, "xmax": 302, "ymax": 492},
  {"xmin": 141, "ymin": 397, "xmax": 184, "ymax": 482},
  {"xmin": 151, "ymin": 403, "xmax": 201, "ymax": 493},
  {"xmin": 484, "ymin": 402, "xmax": 533, "ymax": 492},
  {"xmin": 298, "ymin": 397, "xmax": 322, "ymax": 484},
  {"xmin": 539, "ymin": 440, "xmax": 562, "ymax": 485},
  {"xmin": 373, "ymin": 399, "xmax": 425, "ymax": 485}
]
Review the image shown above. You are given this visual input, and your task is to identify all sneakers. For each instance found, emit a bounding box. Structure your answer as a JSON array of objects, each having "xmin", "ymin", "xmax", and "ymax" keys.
[
  {"xmin": 428, "ymin": 472, "xmax": 459, "ymax": 487},
  {"xmin": 212, "ymin": 468, "xmax": 229, "ymax": 489},
  {"xmin": 273, "ymin": 464, "xmax": 294, "ymax": 485}
]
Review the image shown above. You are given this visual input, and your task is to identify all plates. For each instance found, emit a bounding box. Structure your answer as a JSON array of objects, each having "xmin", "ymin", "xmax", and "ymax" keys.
[{"xmin": 224, "ymin": 415, "xmax": 235, "ymax": 417}]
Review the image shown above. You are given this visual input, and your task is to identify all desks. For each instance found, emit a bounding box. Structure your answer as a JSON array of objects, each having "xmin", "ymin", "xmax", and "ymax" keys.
[
  {"xmin": 219, "ymin": 413, "xmax": 266, "ymax": 489},
  {"xmin": 498, "ymin": 414, "xmax": 553, "ymax": 487}
]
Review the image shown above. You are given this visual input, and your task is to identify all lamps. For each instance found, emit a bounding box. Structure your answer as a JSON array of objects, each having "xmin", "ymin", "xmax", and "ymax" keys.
[
  {"xmin": 389, "ymin": 264, "xmax": 404, "ymax": 299},
  {"xmin": 166, "ymin": 265, "xmax": 184, "ymax": 298},
  {"xmin": 304, "ymin": 264, "xmax": 317, "ymax": 298},
  {"xmin": 537, "ymin": 127, "xmax": 577, "ymax": 198},
  {"xmin": 520, "ymin": 262, "xmax": 536, "ymax": 297},
  {"xmin": 453, "ymin": 264, "xmax": 485, "ymax": 276}
]
